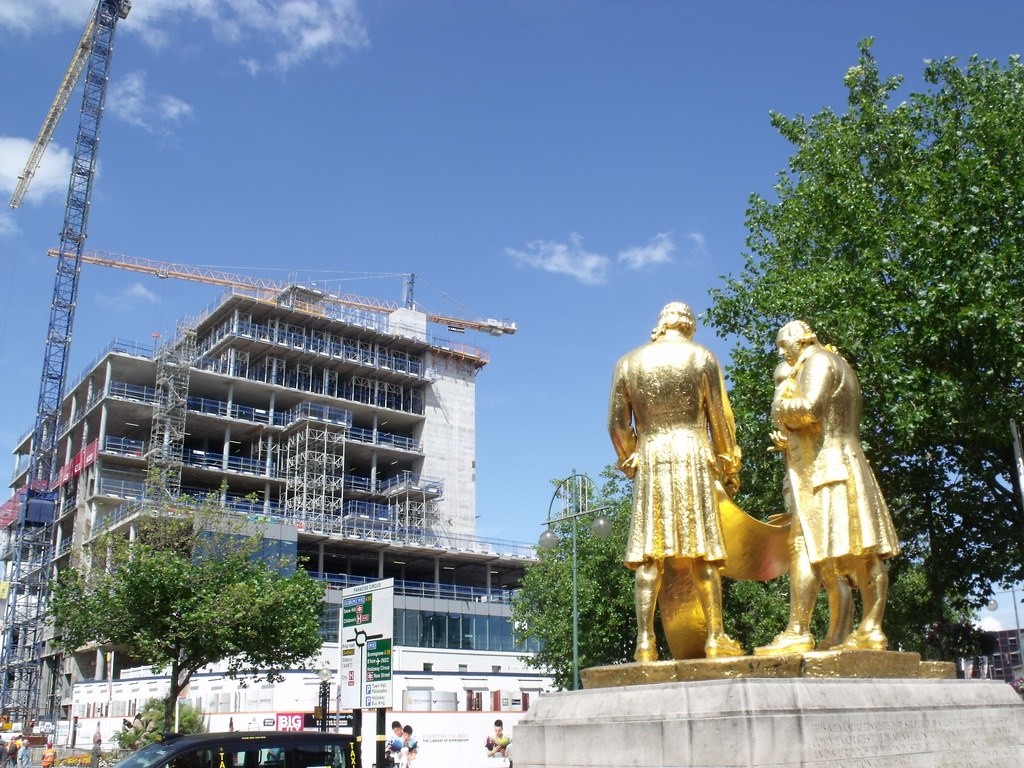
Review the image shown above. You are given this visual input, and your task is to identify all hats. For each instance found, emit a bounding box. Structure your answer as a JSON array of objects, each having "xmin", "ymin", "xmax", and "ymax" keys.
[
  {"xmin": 97, "ymin": 738, "xmax": 102, "ymax": 743},
  {"xmin": 11, "ymin": 736, "xmax": 17, "ymax": 740}
]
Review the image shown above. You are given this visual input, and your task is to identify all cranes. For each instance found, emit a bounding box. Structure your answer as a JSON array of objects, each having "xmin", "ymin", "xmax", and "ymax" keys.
[
  {"xmin": 0, "ymin": 0, "xmax": 131, "ymax": 746},
  {"xmin": 49, "ymin": 244, "xmax": 517, "ymax": 339}
]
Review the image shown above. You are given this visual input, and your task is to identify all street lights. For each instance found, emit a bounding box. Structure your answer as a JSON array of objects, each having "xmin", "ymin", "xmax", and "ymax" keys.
[
  {"xmin": 987, "ymin": 587, "xmax": 1024, "ymax": 670},
  {"xmin": 539, "ymin": 468, "xmax": 615, "ymax": 691},
  {"xmin": 318, "ymin": 668, "xmax": 332, "ymax": 732}
]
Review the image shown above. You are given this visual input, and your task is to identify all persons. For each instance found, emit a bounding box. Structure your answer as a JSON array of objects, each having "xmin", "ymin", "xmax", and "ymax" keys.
[
  {"xmin": 18, "ymin": 740, "xmax": 32, "ymax": 768},
  {"xmin": 609, "ymin": 302, "xmax": 746, "ymax": 659},
  {"xmin": 93, "ymin": 721, "xmax": 101, "ymax": 743},
  {"xmin": 753, "ymin": 320, "xmax": 900, "ymax": 657},
  {"xmin": 486, "ymin": 719, "xmax": 513, "ymax": 768},
  {"xmin": 91, "ymin": 739, "xmax": 101, "ymax": 768},
  {"xmin": 0, "ymin": 734, "xmax": 23, "ymax": 768},
  {"xmin": 385, "ymin": 721, "xmax": 418, "ymax": 768},
  {"xmin": 267, "ymin": 748, "xmax": 281, "ymax": 761},
  {"xmin": 41, "ymin": 742, "xmax": 57, "ymax": 768}
]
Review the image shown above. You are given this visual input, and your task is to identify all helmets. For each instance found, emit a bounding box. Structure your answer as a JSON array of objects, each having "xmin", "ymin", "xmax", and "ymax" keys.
[{"xmin": 47, "ymin": 743, "xmax": 52, "ymax": 747}]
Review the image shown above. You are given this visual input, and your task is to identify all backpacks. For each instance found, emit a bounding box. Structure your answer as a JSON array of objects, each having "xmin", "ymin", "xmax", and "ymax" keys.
[{"xmin": 9, "ymin": 742, "xmax": 17, "ymax": 754}]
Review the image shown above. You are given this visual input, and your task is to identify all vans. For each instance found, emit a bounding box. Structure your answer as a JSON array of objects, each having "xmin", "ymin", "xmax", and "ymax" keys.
[{"xmin": 114, "ymin": 732, "xmax": 363, "ymax": 768}]
